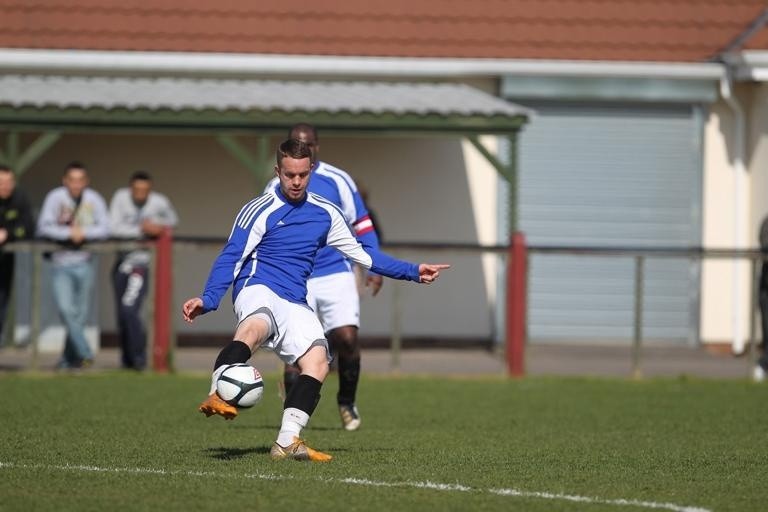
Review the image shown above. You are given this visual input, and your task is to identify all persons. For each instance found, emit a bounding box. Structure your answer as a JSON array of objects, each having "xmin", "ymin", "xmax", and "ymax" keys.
[
  {"xmin": 108, "ymin": 170, "xmax": 177, "ymax": 374},
  {"xmin": 35, "ymin": 159, "xmax": 109, "ymax": 371},
  {"xmin": 180, "ymin": 139, "xmax": 451, "ymax": 463},
  {"xmin": 1, "ymin": 164, "xmax": 34, "ymax": 344},
  {"xmin": 262, "ymin": 120, "xmax": 385, "ymax": 433}
]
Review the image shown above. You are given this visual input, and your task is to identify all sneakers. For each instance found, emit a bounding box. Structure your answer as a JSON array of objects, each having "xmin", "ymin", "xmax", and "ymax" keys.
[
  {"xmin": 339, "ymin": 405, "xmax": 360, "ymax": 430},
  {"xmin": 199, "ymin": 391, "xmax": 237, "ymax": 421},
  {"xmin": 271, "ymin": 436, "xmax": 333, "ymax": 463}
]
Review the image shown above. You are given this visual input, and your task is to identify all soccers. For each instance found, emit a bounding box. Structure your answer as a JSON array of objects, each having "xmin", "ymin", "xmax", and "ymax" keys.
[{"xmin": 217, "ymin": 362, "xmax": 264, "ymax": 407}]
